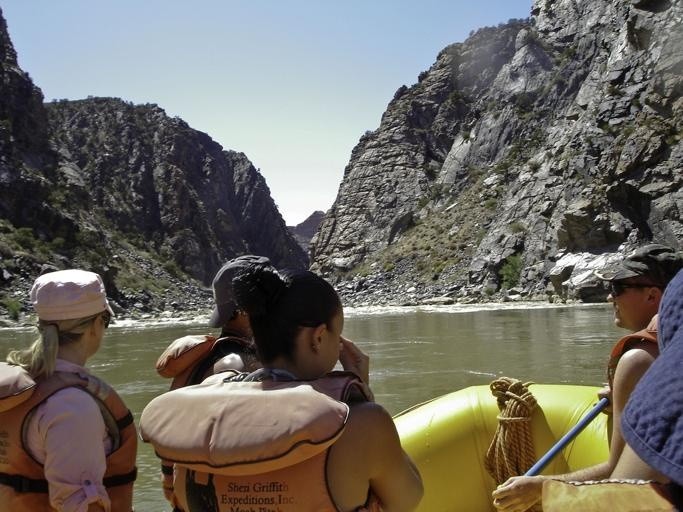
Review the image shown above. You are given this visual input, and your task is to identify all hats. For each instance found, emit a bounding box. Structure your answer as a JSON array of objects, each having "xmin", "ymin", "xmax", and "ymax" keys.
[
  {"xmin": 29, "ymin": 268, "xmax": 117, "ymax": 322},
  {"xmin": 593, "ymin": 243, "xmax": 682, "ymax": 286},
  {"xmin": 206, "ymin": 254, "xmax": 273, "ymax": 329}
]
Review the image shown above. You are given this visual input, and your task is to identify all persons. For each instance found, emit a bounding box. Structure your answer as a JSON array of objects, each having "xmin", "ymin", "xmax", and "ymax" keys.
[
  {"xmin": 488, "ymin": 242, "xmax": 681, "ymax": 512},
  {"xmin": 138, "ymin": 263, "xmax": 424, "ymax": 512},
  {"xmin": 155, "ymin": 252, "xmax": 284, "ymax": 396},
  {"xmin": 602, "ymin": 265, "xmax": 681, "ymax": 485},
  {"xmin": 0, "ymin": 267, "xmax": 138, "ymax": 512}
]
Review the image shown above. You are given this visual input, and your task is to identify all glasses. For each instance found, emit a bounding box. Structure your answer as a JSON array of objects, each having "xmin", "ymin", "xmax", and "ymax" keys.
[
  {"xmin": 92, "ymin": 313, "xmax": 111, "ymax": 329},
  {"xmin": 609, "ymin": 282, "xmax": 661, "ymax": 298}
]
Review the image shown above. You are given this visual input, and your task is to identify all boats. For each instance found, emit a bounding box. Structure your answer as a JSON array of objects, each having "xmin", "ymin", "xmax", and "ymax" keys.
[{"xmin": 344, "ymin": 382, "xmax": 617, "ymax": 512}]
